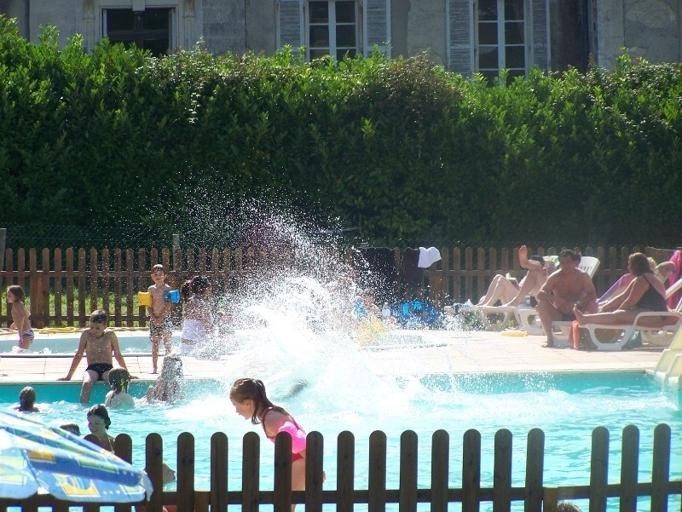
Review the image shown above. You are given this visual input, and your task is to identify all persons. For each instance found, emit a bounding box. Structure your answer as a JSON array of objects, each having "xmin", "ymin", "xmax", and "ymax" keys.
[
  {"xmin": 229, "ymin": 378, "xmax": 325, "ymax": 511},
  {"xmin": 135, "ymin": 463, "xmax": 180, "ymax": 512}
]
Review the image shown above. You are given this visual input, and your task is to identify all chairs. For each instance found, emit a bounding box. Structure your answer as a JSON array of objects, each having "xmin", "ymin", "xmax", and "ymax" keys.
[{"xmin": 456, "ymin": 249, "xmax": 682, "ymax": 354}]
